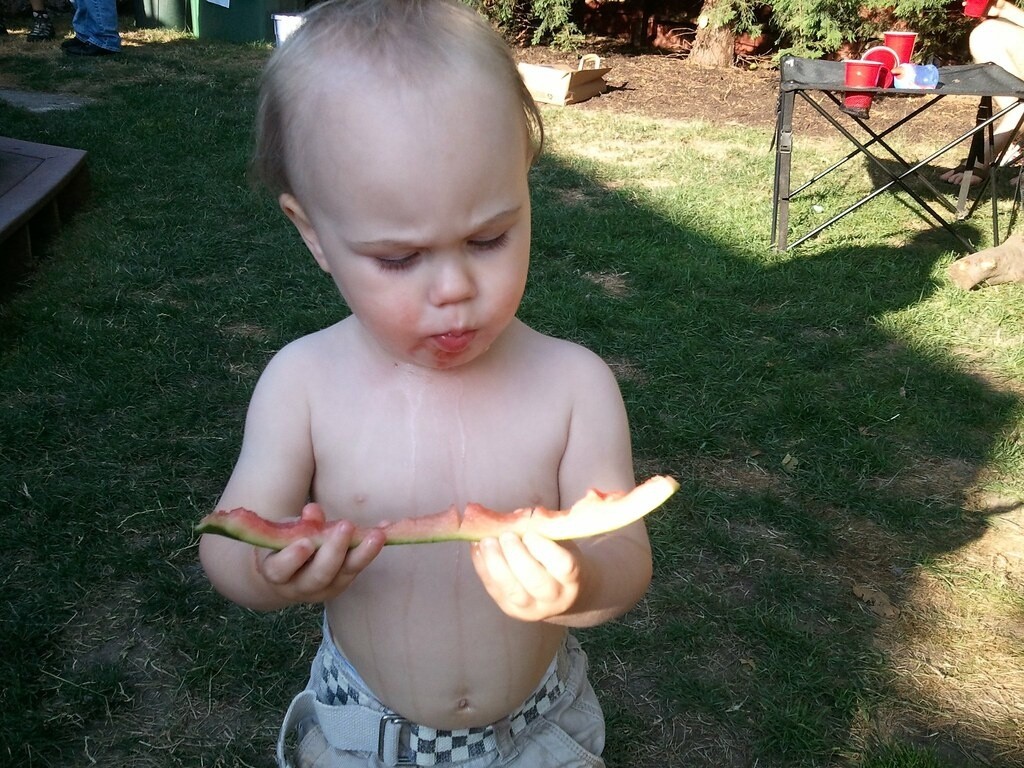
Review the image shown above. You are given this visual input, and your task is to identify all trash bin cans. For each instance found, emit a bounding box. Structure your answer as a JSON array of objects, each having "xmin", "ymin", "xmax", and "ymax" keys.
[{"xmin": 143, "ymin": 0, "xmax": 274, "ymax": 45}]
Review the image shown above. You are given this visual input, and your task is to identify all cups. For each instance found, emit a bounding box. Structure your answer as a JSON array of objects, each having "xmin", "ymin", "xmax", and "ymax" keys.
[
  {"xmin": 891, "ymin": 64, "xmax": 938, "ymax": 89},
  {"xmin": 963, "ymin": 0, "xmax": 990, "ymax": 17},
  {"xmin": 860, "ymin": 45, "xmax": 899, "ymax": 87},
  {"xmin": 844, "ymin": 58, "xmax": 882, "ymax": 108},
  {"xmin": 883, "ymin": 30, "xmax": 919, "ymax": 66}
]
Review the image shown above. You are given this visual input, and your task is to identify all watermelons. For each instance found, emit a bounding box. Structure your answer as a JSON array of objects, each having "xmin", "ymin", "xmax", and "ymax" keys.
[{"xmin": 194, "ymin": 476, "xmax": 679, "ymax": 552}]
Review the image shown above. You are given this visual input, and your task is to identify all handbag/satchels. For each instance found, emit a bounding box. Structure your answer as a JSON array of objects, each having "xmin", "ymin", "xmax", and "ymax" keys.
[{"xmin": 517, "ymin": 52, "xmax": 613, "ymax": 107}]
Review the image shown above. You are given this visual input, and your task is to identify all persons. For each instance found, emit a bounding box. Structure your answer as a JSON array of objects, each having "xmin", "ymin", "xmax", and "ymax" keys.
[
  {"xmin": 27, "ymin": 0, "xmax": 120, "ymax": 55},
  {"xmin": 198, "ymin": 0, "xmax": 650, "ymax": 768}
]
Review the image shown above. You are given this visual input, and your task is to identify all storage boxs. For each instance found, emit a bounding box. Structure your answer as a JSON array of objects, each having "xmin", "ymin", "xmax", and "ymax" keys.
[{"xmin": 519, "ymin": 60, "xmax": 612, "ymax": 106}]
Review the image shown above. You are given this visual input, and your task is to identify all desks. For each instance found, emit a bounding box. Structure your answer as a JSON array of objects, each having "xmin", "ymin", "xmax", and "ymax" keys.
[{"xmin": 770, "ymin": 54, "xmax": 1024, "ymax": 253}]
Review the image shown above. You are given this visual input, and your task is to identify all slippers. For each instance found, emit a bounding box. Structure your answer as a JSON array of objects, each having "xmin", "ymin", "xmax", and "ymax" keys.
[{"xmin": 944, "ymin": 161, "xmax": 990, "ymax": 190}]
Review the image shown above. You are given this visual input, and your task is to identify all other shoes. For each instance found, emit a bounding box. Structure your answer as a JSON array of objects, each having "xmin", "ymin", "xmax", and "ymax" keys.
[
  {"xmin": 64, "ymin": 41, "xmax": 115, "ymax": 57},
  {"xmin": 61, "ymin": 37, "xmax": 84, "ymax": 49},
  {"xmin": 26, "ymin": 10, "xmax": 56, "ymax": 44}
]
